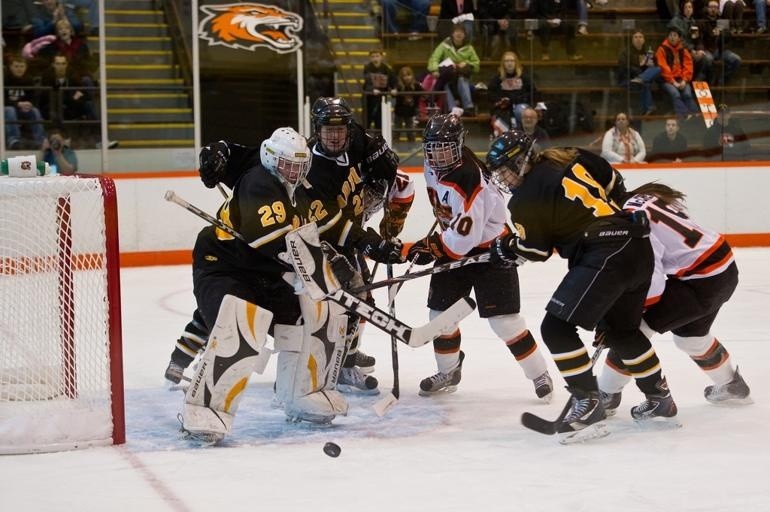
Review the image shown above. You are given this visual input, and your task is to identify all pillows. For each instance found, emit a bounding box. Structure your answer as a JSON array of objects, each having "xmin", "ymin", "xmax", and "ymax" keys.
[
  {"xmin": 420, "ymin": 111, "xmax": 466, "ymax": 171},
  {"xmin": 480, "ymin": 130, "xmax": 534, "ymax": 195},
  {"xmin": 309, "ymin": 95, "xmax": 353, "ymax": 158},
  {"xmin": 259, "ymin": 126, "xmax": 312, "ymax": 189}
]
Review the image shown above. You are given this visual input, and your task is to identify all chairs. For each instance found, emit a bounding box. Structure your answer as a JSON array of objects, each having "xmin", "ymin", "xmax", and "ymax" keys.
[
  {"xmin": 418, "ymin": 349, "xmax": 466, "ymax": 392},
  {"xmin": 599, "ymin": 391, "xmax": 623, "ymax": 410},
  {"xmin": 163, "ymin": 359, "xmax": 184, "ymax": 385},
  {"xmin": 703, "ymin": 366, "xmax": 750, "ymax": 402},
  {"xmin": 353, "ymin": 349, "xmax": 377, "ymax": 367},
  {"xmin": 555, "ymin": 375, "xmax": 608, "ymax": 434},
  {"xmin": 337, "ymin": 365, "xmax": 378, "ymax": 392},
  {"xmin": 629, "ymin": 375, "xmax": 678, "ymax": 421},
  {"xmin": 532, "ymin": 369, "xmax": 554, "ymax": 400}
]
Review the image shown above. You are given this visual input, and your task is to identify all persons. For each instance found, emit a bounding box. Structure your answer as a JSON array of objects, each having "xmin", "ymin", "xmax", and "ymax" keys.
[
  {"xmin": 405, "ymin": 115, "xmax": 554, "ymax": 409},
  {"xmin": 162, "ymin": 97, "xmax": 417, "ymax": 394},
  {"xmin": 595, "ymin": 182, "xmax": 755, "ymax": 417},
  {"xmin": 171, "ymin": 127, "xmax": 349, "ymax": 448},
  {"xmin": 40, "ymin": 53, "xmax": 118, "ymax": 150},
  {"xmin": 484, "ymin": 0, "xmax": 596, "ymax": 61},
  {"xmin": 488, "ymin": 51, "xmax": 548, "ymax": 137},
  {"xmin": 35, "ymin": 20, "xmax": 92, "ymax": 79},
  {"xmin": 487, "ymin": 129, "xmax": 680, "ymax": 446},
  {"xmin": 615, "ymin": 30, "xmax": 661, "ymax": 116},
  {"xmin": 427, "ymin": 23, "xmax": 485, "ymax": 116},
  {"xmin": 437, "ymin": 0, "xmax": 475, "ymax": 46},
  {"xmin": 396, "ymin": 68, "xmax": 423, "ymax": 125},
  {"xmin": 40, "ymin": 130, "xmax": 78, "ymax": 175},
  {"xmin": 360, "ymin": 48, "xmax": 399, "ymax": 130},
  {"xmin": 600, "ymin": 113, "xmax": 647, "ymax": 164},
  {"xmin": 23, "ymin": 0, "xmax": 86, "ymax": 40},
  {"xmin": 378, "ymin": 0, "xmax": 425, "ymax": 33},
  {"xmin": 703, "ymin": 104, "xmax": 751, "ymax": 162},
  {"xmin": 697, "ymin": 0, "xmax": 742, "ymax": 87},
  {"xmin": 655, "ymin": 27, "xmax": 701, "ymax": 115},
  {"xmin": 652, "ymin": 117, "xmax": 690, "ymax": 161},
  {"xmin": 2, "ymin": 55, "xmax": 45, "ymax": 152},
  {"xmin": 670, "ymin": 1, "xmax": 706, "ymax": 82}
]
